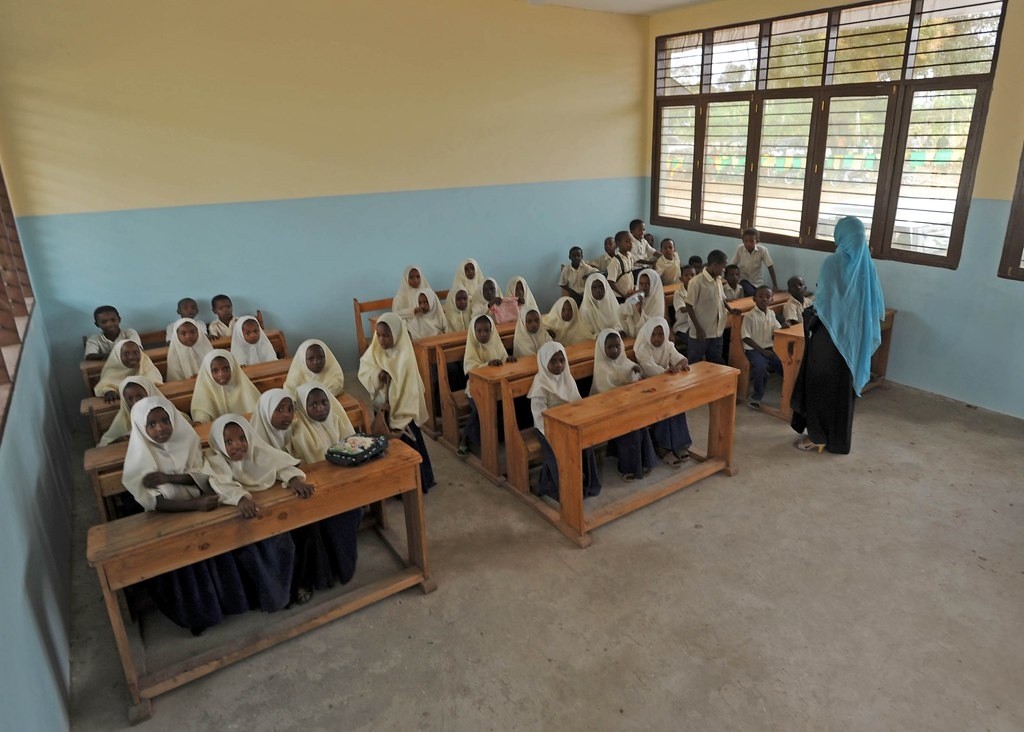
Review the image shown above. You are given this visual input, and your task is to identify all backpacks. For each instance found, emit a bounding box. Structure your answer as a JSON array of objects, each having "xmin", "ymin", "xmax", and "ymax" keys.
[{"xmin": 325, "ymin": 432, "xmax": 389, "ymax": 468}]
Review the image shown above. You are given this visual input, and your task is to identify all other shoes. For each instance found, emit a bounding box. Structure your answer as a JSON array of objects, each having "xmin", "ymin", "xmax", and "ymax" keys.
[
  {"xmin": 623, "ymin": 473, "xmax": 637, "ymax": 482},
  {"xmin": 457, "ymin": 439, "xmax": 469, "ymax": 459},
  {"xmin": 642, "ymin": 467, "xmax": 653, "ymax": 477},
  {"xmin": 748, "ymin": 397, "xmax": 761, "ymax": 411}
]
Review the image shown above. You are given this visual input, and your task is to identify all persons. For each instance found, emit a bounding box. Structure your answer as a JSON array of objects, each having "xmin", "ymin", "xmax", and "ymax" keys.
[
  {"xmin": 94, "ymin": 339, "xmax": 164, "ymax": 405},
  {"xmin": 558, "ymin": 219, "xmax": 814, "ymax": 410},
  {"xmin": 121, "ymin": 382, "xmax": 357, "ymax": 637},
  {"xmin": 282, "ymin": 339, "xmax": 344, "ymax": 397},
  {"xmin": 97, "ymin": 375, "xmax": 193, "ymax": 448},
  {"xmin": 190, "ymin": 349, "xmax": 262, "ymax": 425},
  {"xmin": 231, "ymin": 315, "xmax": 279, "ymax": 369},
  {"xmin": 166, "ymin": 298, "xmax": 220, "ymax": 347},
  {"xmin": 392, "ymin": 258, "xmax": 693, "ymax": 503},
  {"xmin": 84, "ymin": 305, "xmax": 142, "ymax": 361},
  {"xmin": 358, "ymin": 312, "xmax": 435, "ymax": 494},
  {"xmin": 209, "ymin": 294, "xmax": 239, "ymax": 337},
  {"xmin": 167, "ymin": 318, "xmax": 214, "ymax": 381},
  {"xmin": 789, "ymin": 216, "xmax": 885, "ymax": 455}
]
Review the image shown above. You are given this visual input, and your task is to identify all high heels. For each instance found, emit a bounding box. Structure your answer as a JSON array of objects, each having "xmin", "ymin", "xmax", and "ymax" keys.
[{"xmin": 795, "ymin": 436, "xmax": 826, "ymax": 454}]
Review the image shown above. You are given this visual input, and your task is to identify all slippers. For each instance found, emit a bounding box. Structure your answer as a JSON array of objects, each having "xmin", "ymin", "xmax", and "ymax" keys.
[
  {"xmin": 677, "ymin": 447, "xmax": 691, "ymax": 462},
  {"xmin": 656, "ymin": 450, "xmax": 681, "ymax": 468},
  {"xmin": 295, "ymin": 587, "xmax": 312, "ymax": 605}
]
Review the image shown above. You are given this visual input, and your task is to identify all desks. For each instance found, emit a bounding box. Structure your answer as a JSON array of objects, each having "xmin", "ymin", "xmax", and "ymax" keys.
[
  {"xmin": 411, "ymin": 319, "xmax": 515, "ymax": 441},
  {"xmin": 724, "ymin": 289, "xmax": 812, "ymax": 402},
  {"xmin": 467, "ymin": 334, "xmax": 637, "ymax": 477},
  {"xmin": 85, "ymin": 436, "xmax": 434, "ymax": 731},
  {"xmin": 663, "ymin": 284, "xmax": 682, "ymax": 325},
  {"xmin": 773, "ymin": 307, "xmax": 897, "ymax": 422},
  {"xmin": 543, "ymin": 360, "xmax": 740, "ymax": 549},
  {"xmin": 82, "ymin": 326, "xmax": 289, "ymax": 398},
  {"xmin": 84, "ymin": 390, "xmax": 361, "ymax": 524},
  {"xmin": 80, "ymin": 357, "xmax": 292, "ymax": 443}
]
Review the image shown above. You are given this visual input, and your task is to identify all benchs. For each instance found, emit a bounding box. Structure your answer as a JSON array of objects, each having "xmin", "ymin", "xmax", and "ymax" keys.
[{"xmin": 81, "ymin": 231, "xmax": 900, "ymax": 728}]
